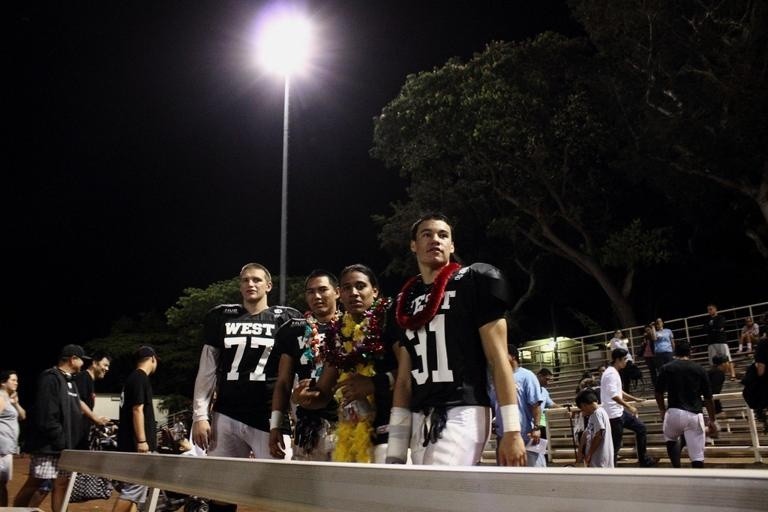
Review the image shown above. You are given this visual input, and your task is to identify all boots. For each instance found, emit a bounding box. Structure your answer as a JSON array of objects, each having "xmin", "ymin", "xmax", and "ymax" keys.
[
  {"xmin": 691, "ymin": 461, "xmax": 704, "ymax": 468},
  {"xmin": 665, "ymin": 440, "xmax": 682, "ymax": 468}
]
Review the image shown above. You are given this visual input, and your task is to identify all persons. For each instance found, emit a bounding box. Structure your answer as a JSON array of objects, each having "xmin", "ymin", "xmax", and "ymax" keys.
[
  {"xmin": 680, "ymin": 353, "xmax": 730, "ymax": 452},
  {"xmin": 174, "ymin": 438, "xmax": 207, "ymax": 456},
  {"xmin": 193, "ymin": 263, "xmax": 304, "ymax": 512},
  {"xmin": 112, "ymin": 347, "xmax": 157, "ymax": 512},
  {"xmin": 386, "ymin": 213, "xmax": 526, "ymax": 466},
  {"xmin": 736, "ymin": 317, "xmax": 759, "ymax": 352},
  {"xmin": 740, "ymin": 341, "xmax": 768, "ymax": 431},
  {"xmin": 14, "ymin": 345, "xmax": 83, "ymax": 512},
  {"xmin": 490, "ymin": 344, "xmax": 544, "ymax": 466},
  {"xmin": 269, "ymin": 270, "xmax": 344, "ymax": 461},
  {"xmin": 655, "ymin": 342, "xmax": 720, "ymax": 469},
  {"xmin": 605, "ymin": 330, "xmax": 633, "ymax": 394},
  {"xmin": 641, "ymin": 324, "xmax": 658, "ymax": 385},
  {"xmin": 649, "ymin": 318, "xmax": 674, "ymax": 370},
  {"xmin": 575, "ymin": 391, "xmax": 614, "ymax": 468},
  {"xmin": 601, "ymin": 348, "xmax": 660, "ymax": 467},
  {"xmin": 0, "ymin": 371, "xmax": 25, "ymax": 507},
  {"xmin": 293, "ymin": 265, "xmax": 412, "ymax": 464},
  {"xmin": 703, "ymin": 305, "xmax": 736, "ymax": 381},
  {"xmin": 28, "ymin": 350, "xmax": 109, "ymax": 507},
  {"xmin": 536, "ymin": 369, "xmax": 573, "ymax": 466}
]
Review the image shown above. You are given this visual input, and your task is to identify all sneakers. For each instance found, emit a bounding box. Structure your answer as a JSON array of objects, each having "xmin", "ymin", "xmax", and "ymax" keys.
[
  {"xmin": 642, "ymin": 455, "xmax": 659, "ymax": 465},
  {"xmin": 747, "ymin": 348, "xmax": 752, "ymax": 352},
  {"xmin": 731, "ymin": 377, "xmax": 741, "ymax": 382},
  {"xmin": 736, "ymin": 349, "xmax": 741, "ymax": 352}
]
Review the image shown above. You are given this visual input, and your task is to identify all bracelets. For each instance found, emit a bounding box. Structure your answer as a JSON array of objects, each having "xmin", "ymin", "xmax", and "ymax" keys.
[{"xmin": 136, "ymin": 440, "xmax": 147, "ymax": 443}]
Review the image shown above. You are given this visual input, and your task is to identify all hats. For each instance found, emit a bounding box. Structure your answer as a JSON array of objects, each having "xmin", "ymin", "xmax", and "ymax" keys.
[
  {"xmin": 62, "ymin": 345, "xmax": 92, "ymax": 359},
  {"xmin": 137, "ymin": 346, "xmax": 162, "ymax": 365}
]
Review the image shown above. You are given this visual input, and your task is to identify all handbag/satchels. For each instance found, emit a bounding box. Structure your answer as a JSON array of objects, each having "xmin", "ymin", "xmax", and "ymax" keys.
[{"xmin": 525, "ymin": 437, "xmax": 547, "ymax": 467}]
